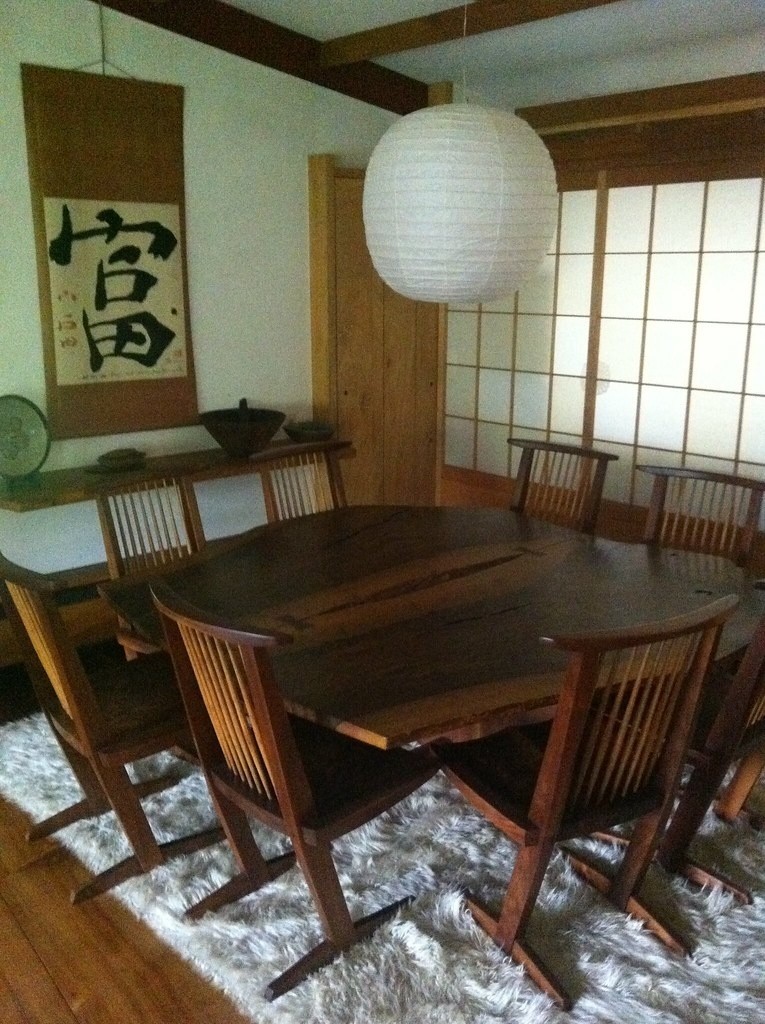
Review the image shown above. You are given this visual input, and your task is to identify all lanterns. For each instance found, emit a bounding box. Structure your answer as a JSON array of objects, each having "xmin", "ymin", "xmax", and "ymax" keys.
[{"xmin": 361, "ymin": 104, "xmax": 557, "ymax": 304}]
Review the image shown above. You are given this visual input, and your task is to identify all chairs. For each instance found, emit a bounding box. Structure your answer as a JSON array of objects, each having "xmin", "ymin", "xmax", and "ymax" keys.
[{"xmin": 0, "ymin": 438, "xmax": 765, "ymax": 1012}]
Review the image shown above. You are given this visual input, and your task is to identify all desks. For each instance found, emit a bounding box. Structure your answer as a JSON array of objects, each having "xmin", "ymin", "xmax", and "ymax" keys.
[
  {"xmin": 96, "ymin": 503, "xmax": 765, "ymax": 789},
  {"xmin": 0, "ymin": 430, "xmax": 356, "ymax": 674}
]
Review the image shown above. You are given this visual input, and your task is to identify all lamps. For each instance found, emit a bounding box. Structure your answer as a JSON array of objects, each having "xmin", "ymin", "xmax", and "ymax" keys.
[{"xmin": 364, "ymin": 0, "xmax": 559, "ymax": 305}]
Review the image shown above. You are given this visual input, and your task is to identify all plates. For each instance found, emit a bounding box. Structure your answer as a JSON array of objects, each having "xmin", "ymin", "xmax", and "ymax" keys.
[
  {"xmin": 0, "ymin": 394, "xmax": 51, "ymax": 479},
  {"xmin": 283, "ymin": 421, "xmax": 335, "ymax": 443}
]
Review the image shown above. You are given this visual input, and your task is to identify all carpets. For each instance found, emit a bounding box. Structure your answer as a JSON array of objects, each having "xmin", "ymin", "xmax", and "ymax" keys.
[{"xmin": 0, "ymin": 705, "xmax": 765, "ymax": 1024}]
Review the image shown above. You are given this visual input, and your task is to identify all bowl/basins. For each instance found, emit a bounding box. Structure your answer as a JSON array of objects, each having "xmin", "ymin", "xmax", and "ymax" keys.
[{"xmin": 200, "ymin": 408, "xmax": 286, "ymax": 458}]
